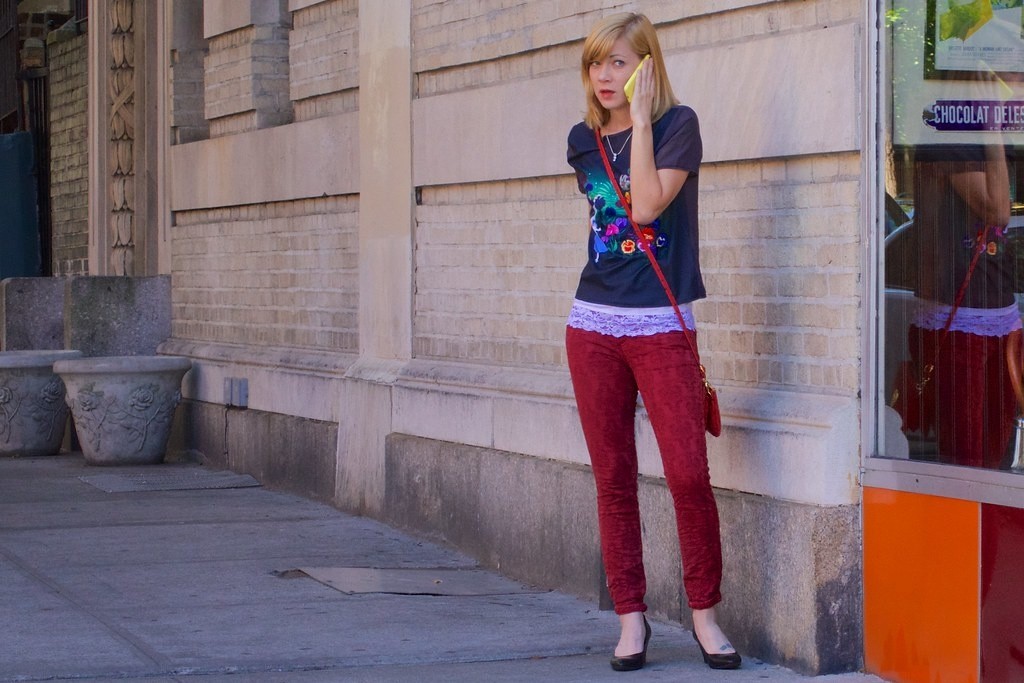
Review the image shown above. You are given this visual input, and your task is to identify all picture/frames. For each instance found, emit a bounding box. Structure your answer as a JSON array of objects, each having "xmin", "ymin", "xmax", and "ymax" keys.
[{"xmin": 923, "ymin": 0, "xmax": 1024, "ymax": 84}]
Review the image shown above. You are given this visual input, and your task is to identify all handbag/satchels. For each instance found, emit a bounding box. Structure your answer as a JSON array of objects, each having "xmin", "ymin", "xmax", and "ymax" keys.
[
  {"xmin": 706, "ymin": 381, "xmax": 723, "ymax": 437},
  {"xmin": 891, "ymin": 361, "xmax": 922, "ymax": 434}
]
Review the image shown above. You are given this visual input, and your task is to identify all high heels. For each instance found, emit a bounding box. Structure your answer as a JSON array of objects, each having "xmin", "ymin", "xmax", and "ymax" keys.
[
  {"xmin": 692, "ymin": 624, "xmax": 741, "ymax": 669},
  {"xmin": 610, "ymin": 614, "xmax": 652, "ymax": 670}
]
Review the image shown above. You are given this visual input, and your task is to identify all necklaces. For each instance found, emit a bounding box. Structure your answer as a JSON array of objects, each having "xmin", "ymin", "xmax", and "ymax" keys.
[{"xmin": 603, "ymin": 126, "xmax": 633, "ymax": 161}]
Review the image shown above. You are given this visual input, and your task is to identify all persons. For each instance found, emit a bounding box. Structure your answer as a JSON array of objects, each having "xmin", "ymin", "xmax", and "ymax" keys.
[
  {"xmin": 565, "ymin": 13, "xmax": 742, "ymax": 673},
  {"xmin": 892, "ymin": 18, "xmax": 1022, "ymax": 471}
]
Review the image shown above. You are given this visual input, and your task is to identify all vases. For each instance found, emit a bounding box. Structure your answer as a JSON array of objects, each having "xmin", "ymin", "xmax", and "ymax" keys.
[
  {"xmin": 0, "ymin": 350, "xmax": 83, "ymax": 455},
  {"xmin": 53, "ymin": 356, "xmax": 193, "ymax": 467}
]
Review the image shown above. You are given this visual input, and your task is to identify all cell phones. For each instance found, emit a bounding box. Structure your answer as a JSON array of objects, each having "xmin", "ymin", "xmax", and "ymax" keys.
[
  {"xmin": 977, "ymin": 59, "xmax": 1013, "ymax": 104},
  {"xmin": 624, "ymin": 54, "xmax": 651, "ymax": 105}
]
